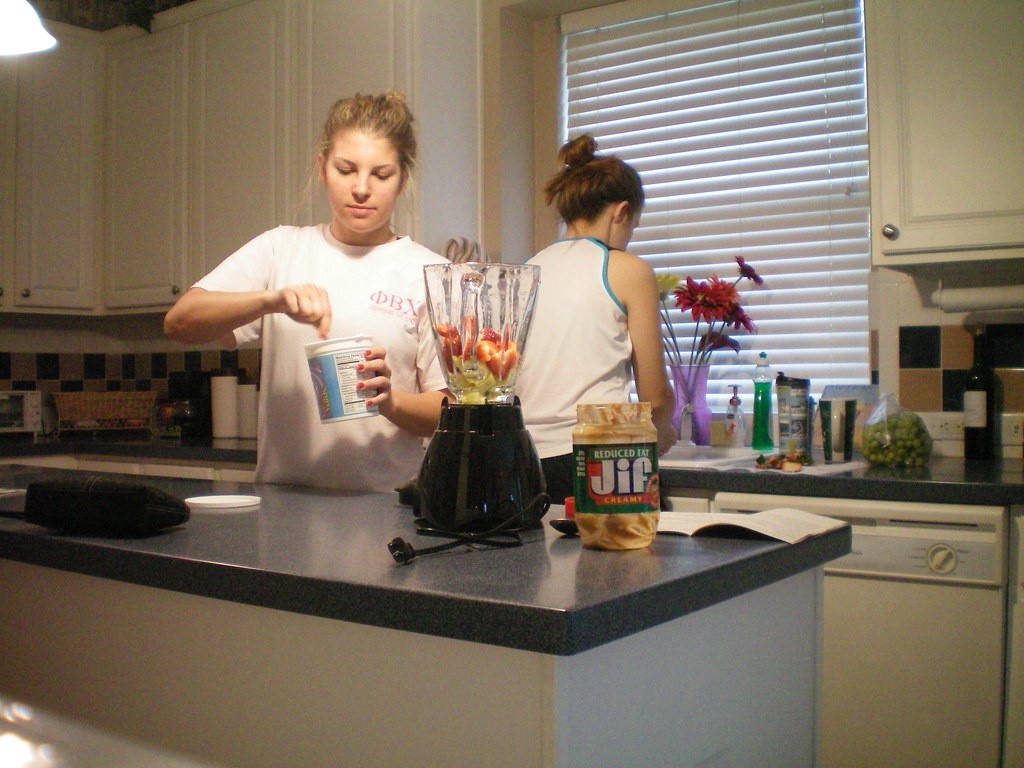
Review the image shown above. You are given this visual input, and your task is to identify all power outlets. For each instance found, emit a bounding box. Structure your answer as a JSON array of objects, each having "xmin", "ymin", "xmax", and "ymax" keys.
[
  {"xmin": 1001, "ymin": 414, "xmax": 1023, "ymax": 446},
  {"xmin": 929, "ymin": 413, "xmax": 962, "ymax": 440}
]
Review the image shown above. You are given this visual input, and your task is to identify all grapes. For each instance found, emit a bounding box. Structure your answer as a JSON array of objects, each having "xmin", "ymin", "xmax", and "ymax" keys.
[{"xmin": 861, "ymin": 411, "xmax": 934, "ymax": 469}]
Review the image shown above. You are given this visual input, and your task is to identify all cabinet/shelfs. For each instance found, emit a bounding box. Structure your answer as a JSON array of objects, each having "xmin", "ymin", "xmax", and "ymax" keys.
[
  {"xmin": 863, "ymin": 0, "xmax": 1024, "ymax": 270},
  {"xmin": 0, "ymin": 0, "xmax": 483, "ymax": 316}
]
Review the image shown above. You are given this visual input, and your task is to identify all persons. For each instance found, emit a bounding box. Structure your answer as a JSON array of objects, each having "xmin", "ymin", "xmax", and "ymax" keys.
[
  {"xmin": 163, "ymin": 91, "xmax": 463, "ymax": 493},
  {"xmin": 505, "ymin": 135, "xmax": 678, "ymax": 505}
]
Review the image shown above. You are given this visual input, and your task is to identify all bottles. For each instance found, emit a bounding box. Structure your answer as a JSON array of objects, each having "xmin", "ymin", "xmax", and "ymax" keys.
[
  {"xmin": 774, "ymin": 372, "xmax": 879, "ymax": 464},
  {"xmin": 752, "ymin": 352, "xmax": 775, "ymax": 451},
  {"xmin": 962, "ymin": 324, "xmax": 1003, "ymax": 476}
]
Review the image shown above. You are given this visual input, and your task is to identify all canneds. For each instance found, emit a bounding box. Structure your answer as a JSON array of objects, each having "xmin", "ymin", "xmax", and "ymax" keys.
[{"xmin": 568, "ymin": 402, "xmax": 659, "ymax": 550}]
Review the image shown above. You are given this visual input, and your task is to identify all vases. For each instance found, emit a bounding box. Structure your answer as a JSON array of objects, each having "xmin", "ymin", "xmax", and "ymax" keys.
[{"xmin": 670, "ymin": 364, "xmax": 713, "ymax": 445}]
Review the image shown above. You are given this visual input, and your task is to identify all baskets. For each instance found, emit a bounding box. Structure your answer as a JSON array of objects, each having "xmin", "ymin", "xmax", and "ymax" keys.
[{"xmin": 53, "ymin": 391, "xmax": 158, "ymax": 421}]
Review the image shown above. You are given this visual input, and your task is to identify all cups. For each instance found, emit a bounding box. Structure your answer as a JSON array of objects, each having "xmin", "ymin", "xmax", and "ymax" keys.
[
  {"xmin": 818, "ymin": 399, "xmax": 860, "ymax": 464},
  {"xmin": 304, "ymin": 333, "xmax": 379, "ymax": 423},
  {"xmin": 211, "ymin": 377, "xmax": 261, "ymax": 440}
]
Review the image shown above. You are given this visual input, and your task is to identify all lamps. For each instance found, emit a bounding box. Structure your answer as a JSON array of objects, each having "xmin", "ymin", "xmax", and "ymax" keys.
[
  {"xmin": 0, "ymin": 1, "xmax": 57, "ymax": 57},
  {"xmin": 655, "ymin": 255, "xmax": 763, "ymax": 363}
]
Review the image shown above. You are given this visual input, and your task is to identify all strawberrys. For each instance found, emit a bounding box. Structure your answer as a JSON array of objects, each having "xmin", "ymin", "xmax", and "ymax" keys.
[{"xmin": 434, "ymin": 315, "xmax": 519, "ymax": 380}]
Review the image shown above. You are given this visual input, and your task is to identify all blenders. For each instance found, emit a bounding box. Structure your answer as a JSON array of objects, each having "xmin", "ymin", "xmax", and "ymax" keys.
[{"xmin": 412, "ymin": 261, "xmax": 550, "ymax": 537}]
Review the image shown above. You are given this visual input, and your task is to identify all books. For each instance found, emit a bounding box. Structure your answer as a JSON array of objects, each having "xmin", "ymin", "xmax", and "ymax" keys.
[{"xmin": 655, "ymin": 499, "xmax": 849, "ymax": 544}]
